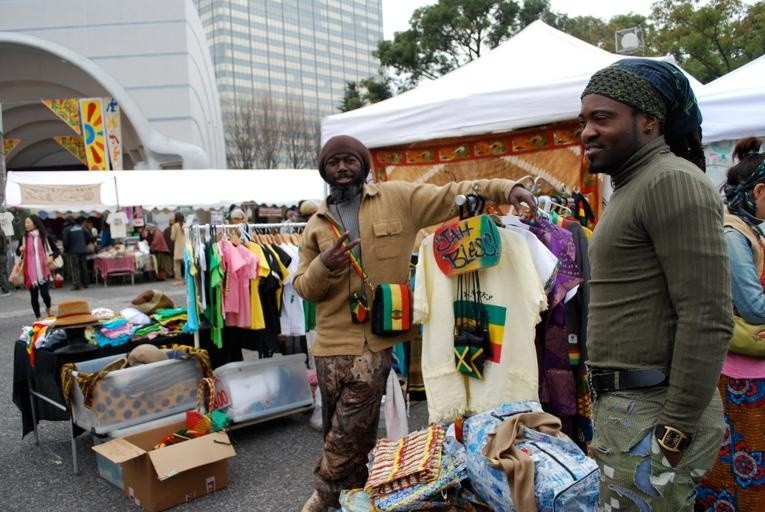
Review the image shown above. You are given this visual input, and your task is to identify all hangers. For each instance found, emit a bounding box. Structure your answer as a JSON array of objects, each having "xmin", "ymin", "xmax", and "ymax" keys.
[
  {"xmin": 172, "ymin": 218, "xmax": 314, "ymax": 258},
  {"xmin": 453, "ymin": 187, "xmax": 582, "ymax": 239}
]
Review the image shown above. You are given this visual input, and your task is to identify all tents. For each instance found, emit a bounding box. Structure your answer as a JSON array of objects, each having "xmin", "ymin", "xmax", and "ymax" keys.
[
  {"xmin": 322, "ymin": 17, "xmax": 630, "ymax": 212},
  {"xmin": 2, "ymin": 167, "xmax": 369, "ymax": 215},
  {"xmin": 682, "ymin": 51, "xmax": 763, "ymax": 190}
]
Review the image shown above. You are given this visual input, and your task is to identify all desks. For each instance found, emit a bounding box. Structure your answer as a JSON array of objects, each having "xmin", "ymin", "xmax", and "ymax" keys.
[
  {"xmin": 8, "ymin": 317, "xmax": 220, "ymax": 476},
  {"xmin": 95, "ymin": 243, "xmax": 153, "ymax": 286}
]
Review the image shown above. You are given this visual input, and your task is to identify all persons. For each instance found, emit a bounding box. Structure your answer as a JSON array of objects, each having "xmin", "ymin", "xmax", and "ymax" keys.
[
  {"xmin": 228, "ymin": 198, "xmax": 317, "ymax": 227},
  {"xmin": 0, "ymin": 208, "xmax": 98, "ymax": 321},
  {"xmin": 293, "ymin": 134, "xmax": 541, "ymax": 512},
  {"xmin": 141, "ymin": 213, "xmax": 189, "ymax": 285},
  {"xmin": 688, "ymin": 137, "xmax": 765, "ymax": 511},
  {"xmin": 580, "ymin": 59, "xmax": 732, "ymax": 510}
]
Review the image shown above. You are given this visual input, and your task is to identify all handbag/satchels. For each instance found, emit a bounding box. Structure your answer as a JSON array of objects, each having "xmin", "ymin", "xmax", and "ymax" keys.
[
  {"xmin": 8, "ymin": 258, "xmax": 24, "ymax": 286},
  {"xmin": 453, "ymin": 326, "xmax": 492, "ymax": 380},
  {"xmin": 338, "ymin": 397, "xmax": 600, "ymax": 512},
  {"xmin": 46, "ymin": 254, "xmax": 63, "ymax": 270},
  {"xmin": 371, "ymin": 281, "xmax": 412, "ymax": 337},
  {"xmin": 729, "ymin": 315, "xmax": 764, "ymax": 355}
]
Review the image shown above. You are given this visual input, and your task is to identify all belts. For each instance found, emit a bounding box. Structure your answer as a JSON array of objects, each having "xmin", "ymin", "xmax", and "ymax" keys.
[{"xmin": 586, "ymin": 370, "xmax": 669, "ymax": 396}]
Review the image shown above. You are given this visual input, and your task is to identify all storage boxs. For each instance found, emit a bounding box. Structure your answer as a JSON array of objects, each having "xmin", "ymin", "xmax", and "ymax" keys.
[
  {"xmin": 212, "ymin": 351, "xmax": 314, "ymax": 423},
  {"xmin": 90, "ymin": 423, "xmax": 237, "ymax": 511},
  {"xmin": 93, "ymin": 407, "xmax": 207, "ymax": 488},
  {"xmin": 67, "ymin": 348, "xmax": 204, "ymax": 436}
]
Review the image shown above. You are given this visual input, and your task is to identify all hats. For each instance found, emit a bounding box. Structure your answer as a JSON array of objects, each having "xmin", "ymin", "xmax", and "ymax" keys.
[
  {"xmin": 53, "ymin": 301, "xmax": 101, "ymax": 329},
  {"xmin": 300, "ymin": 201, "xmax": 317, "ymax": 214},
  {"xmin": 319, "ymin": 137, "xmax": 371, "ymax": 178}
]
